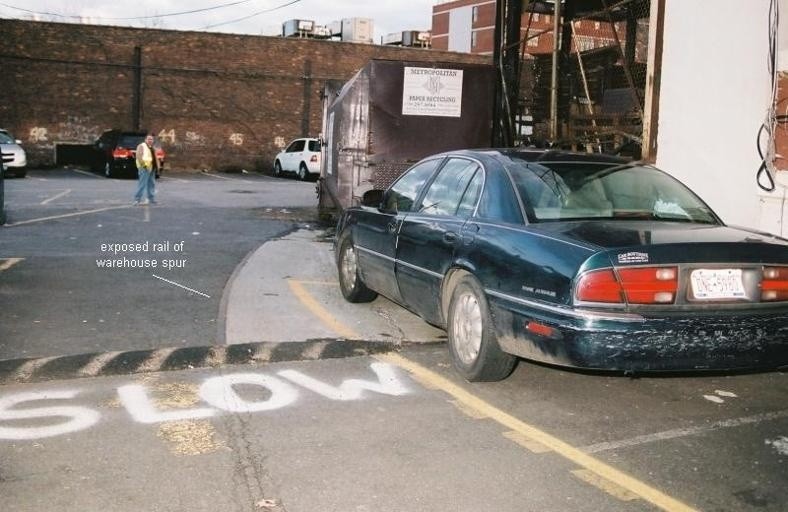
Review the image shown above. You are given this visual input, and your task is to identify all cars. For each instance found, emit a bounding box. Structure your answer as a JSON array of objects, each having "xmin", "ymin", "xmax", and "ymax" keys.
[
  {"xmin": 273, "ymin": 137, "xmax": 321, "ymax": 181},
  {"xmin": 333, "ymin": 147, "xmax": 788, "ymax": 383},
  {"xmin": 94, "ymin": 129, "xmax": 166, "ymax": 179},
  {"xmin": 0, "ymin": 128, "xmax": 28, "ymax": 178}
]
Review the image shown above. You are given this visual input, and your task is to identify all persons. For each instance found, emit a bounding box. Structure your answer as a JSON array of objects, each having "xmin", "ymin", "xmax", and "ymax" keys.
[{"xmin": 135, "ymin": 133, "xmax": 159, "ymax": 205}]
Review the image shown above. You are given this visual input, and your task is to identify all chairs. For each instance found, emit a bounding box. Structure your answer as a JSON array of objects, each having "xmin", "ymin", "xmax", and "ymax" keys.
[{"xmin": 522, "ymin": 171, "xmax": 612, "ymax": 210}]
